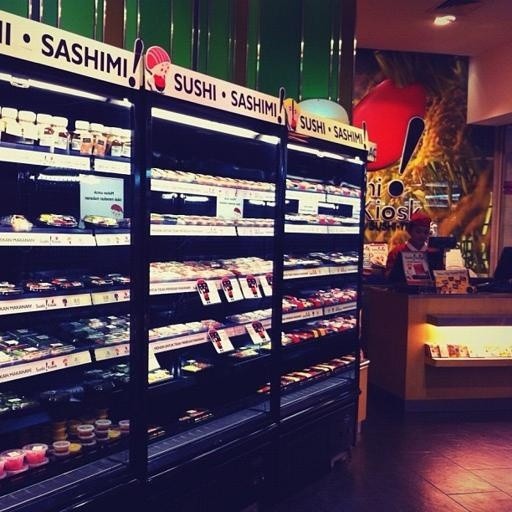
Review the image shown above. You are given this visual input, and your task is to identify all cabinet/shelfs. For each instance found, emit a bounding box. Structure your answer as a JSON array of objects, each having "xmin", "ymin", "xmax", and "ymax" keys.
[
  {"xmin": 142, "ymin": 105, "xmax": 278, "ymax": 466},
  {"xmin": 0, "ymin": 83, "xmax": 140, "ymax": 493},
  {"xmin": 279, "ymin": 149, "xmax": 366, "ymax": 413}
]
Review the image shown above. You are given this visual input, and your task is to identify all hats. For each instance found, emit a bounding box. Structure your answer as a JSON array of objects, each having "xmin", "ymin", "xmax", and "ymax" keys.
[{"xmin": 406, "ymin": 212, "xmax": 432, "ymax": 224}]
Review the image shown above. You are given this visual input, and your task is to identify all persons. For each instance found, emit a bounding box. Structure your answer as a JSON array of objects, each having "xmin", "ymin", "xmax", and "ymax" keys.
[{"xmin": 386, "ymin": 212, "xmax": 440, "ymax": 284}]
